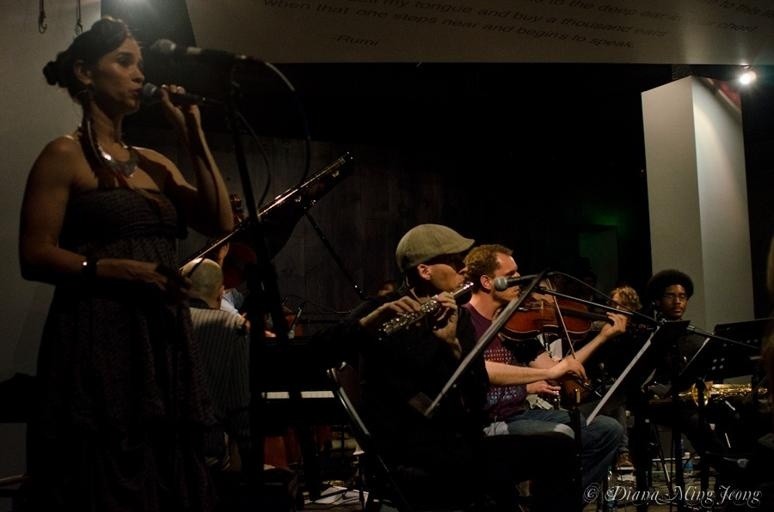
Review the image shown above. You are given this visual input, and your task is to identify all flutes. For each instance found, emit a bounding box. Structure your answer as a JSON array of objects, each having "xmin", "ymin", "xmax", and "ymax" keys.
[{"xmin": 375, "ymin": 280, "xmax": 476, "ymax": 341}]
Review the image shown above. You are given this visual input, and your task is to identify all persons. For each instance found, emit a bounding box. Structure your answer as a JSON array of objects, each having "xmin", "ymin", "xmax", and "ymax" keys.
[
  {"xmin": 15, "ymin": 13, "xmax": 237, "ymax": 511},
  {"xmin": 178, "ymin": 220, "xmax": 740, "ymax": 510}
]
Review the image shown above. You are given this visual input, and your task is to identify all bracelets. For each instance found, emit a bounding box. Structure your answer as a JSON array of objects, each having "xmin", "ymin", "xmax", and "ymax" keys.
[{"xmin": 80, "ymin": 254, "xmax": 101, "ymax": 290}]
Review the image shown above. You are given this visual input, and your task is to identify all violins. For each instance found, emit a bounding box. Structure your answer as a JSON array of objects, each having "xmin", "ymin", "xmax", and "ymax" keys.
[{"xmin": 495, "ymin": 291, "xmax": 663, "ymax": 346}]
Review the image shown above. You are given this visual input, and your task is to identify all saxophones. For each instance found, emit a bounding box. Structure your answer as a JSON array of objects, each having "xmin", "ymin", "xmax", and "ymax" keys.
[{"xmin": 649, "ymin": 381, "xmax": 769, "ymax": 411}]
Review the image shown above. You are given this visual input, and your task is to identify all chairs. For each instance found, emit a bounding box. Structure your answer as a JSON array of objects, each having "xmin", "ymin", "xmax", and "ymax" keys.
[{"xmin": 326, "ymin": 361, "xmax": 525, "ymax": 512}]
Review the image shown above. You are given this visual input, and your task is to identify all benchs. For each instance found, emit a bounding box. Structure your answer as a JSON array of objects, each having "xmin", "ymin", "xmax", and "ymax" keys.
[{"xmin": 0, "ymin": 372, "xmax": 37, "ymax": 512}]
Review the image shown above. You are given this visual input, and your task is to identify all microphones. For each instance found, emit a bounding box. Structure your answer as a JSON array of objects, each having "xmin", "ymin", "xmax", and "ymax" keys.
[
  {"xmin": 150, "ymin": 38, "xmax": 259, "ymax": 63},
  {"xmin": 493, "ymin": 271, "xmax": 560, "ymax": 291},
  {"xmin": 140, "ymin": 81, "xmax": 224, "ymax": 107}
]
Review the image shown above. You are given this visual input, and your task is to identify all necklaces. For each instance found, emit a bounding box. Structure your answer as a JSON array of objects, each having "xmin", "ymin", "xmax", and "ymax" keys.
[{"xmin": 96, "ymin": 135, "xmax": 139, "ymax": 179}]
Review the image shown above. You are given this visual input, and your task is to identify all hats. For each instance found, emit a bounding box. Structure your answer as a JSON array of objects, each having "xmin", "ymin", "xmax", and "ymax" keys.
[{"xmin": 395, "ymin": 224, "xmax": 474, "ymax": 272}]
